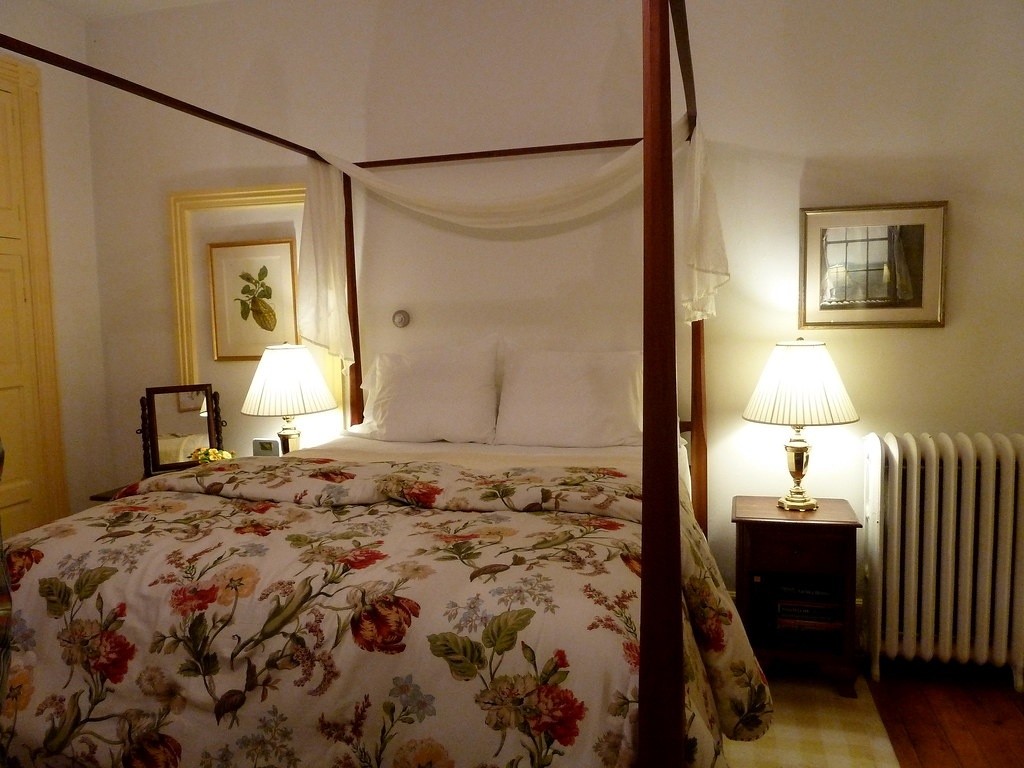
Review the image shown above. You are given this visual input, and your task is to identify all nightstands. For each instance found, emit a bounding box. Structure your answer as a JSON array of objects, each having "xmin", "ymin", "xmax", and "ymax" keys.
[{"xmin": 730, "ymin": 493, "xmax": 865, "ymax": 701}]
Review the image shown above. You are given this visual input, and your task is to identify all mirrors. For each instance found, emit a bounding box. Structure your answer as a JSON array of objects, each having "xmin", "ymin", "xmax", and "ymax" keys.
[{"xmin": 136, "ymin": 383, "xmax": 228, "ymax": 482}]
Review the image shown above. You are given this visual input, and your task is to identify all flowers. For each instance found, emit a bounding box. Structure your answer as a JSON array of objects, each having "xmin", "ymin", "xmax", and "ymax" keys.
[{"xmin": 185, "ymin": 447, "xmax": 236, "ymax": 464}]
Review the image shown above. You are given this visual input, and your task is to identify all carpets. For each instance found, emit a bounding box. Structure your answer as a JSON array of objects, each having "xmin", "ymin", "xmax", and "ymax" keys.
[{"xmin": 714, "ymin": 683, "xmax": 900, "ymax": 768}]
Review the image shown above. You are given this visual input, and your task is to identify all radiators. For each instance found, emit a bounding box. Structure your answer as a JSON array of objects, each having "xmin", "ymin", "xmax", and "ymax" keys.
[{"xmin": 867, "ymin": 430, "xmax": 1024, "ymax": 692}]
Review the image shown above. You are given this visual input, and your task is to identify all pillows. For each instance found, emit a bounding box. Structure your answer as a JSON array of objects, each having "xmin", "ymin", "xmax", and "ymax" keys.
[
  {"xmin": 363, "ymin": 333, "xmax": 499, "ymax": 443},
  {"xmin": 497, "ymin": 350, "xmax": 641, "ymax": 448}
]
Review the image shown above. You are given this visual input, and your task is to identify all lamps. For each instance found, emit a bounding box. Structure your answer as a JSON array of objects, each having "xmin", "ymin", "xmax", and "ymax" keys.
[
  {"xmin": 741, "ymin": 336, "xmax": 856, "ymax": 510},
  {"xmin": 242, "ymin": 341, "xmax": 340, "ymax": 454}
]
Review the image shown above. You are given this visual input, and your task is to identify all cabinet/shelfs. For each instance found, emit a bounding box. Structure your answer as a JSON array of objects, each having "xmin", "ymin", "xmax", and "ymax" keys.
[{"xmin": 1, "ymin": 53, "xmax": 68, "ymax": 542}]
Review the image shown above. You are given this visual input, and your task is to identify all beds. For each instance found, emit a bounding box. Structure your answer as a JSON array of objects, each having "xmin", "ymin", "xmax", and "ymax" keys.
[{"xmin": 0, "ymin": 1, "xmax": 774, "ymax": 768}]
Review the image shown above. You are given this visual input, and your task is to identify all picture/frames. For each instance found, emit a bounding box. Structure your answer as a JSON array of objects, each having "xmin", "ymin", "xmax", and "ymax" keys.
[
  {"xmin": 206, "ymin": 236, "xmax": 299, "ymax": 362},
  {"xmin": 797, "ymin": 200, "xmax": 949, "ymax": 330}
]
